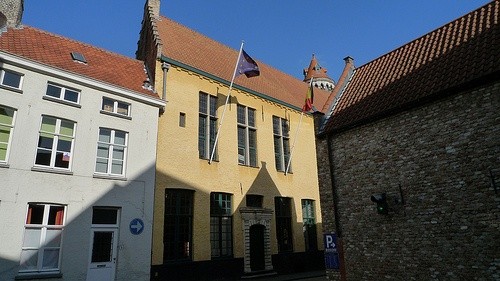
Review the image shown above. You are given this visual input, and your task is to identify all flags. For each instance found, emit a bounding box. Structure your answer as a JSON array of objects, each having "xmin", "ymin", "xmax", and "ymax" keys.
[
  {"xmin": 234, "ymin": 47, "xmax": 260, "ymax": 78},
  {"xmin": 301, "ymin": 80, "xmax": 317, "ymax": 113}
]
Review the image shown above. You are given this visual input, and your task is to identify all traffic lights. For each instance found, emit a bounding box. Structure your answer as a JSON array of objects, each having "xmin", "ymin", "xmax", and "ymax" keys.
[{"xmin": 376, "ymin": 198, "xmax": 387, "ymax": 215}]
